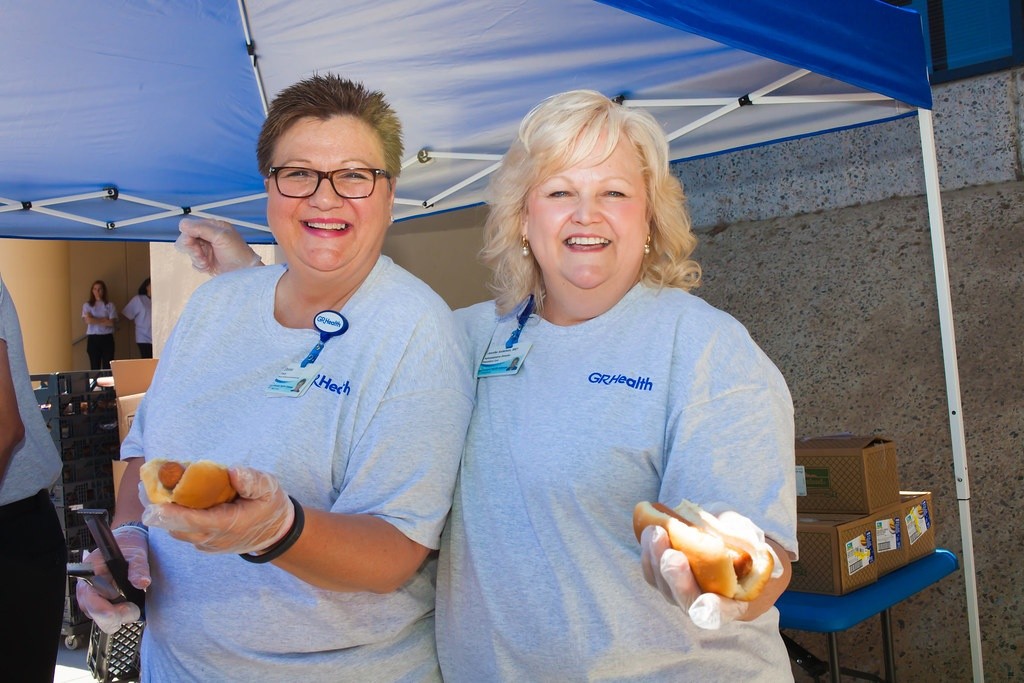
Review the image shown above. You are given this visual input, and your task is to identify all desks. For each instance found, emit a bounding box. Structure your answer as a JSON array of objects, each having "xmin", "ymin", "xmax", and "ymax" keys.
[{"xmin": 764, "ymin": 546, "xmax": 959, "ymax": 683}]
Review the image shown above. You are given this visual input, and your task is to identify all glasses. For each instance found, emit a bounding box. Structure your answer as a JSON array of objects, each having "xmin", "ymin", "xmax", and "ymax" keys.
[{"xmin": 268, "ymin": 165, "xmax": 390, "ymax": 199}]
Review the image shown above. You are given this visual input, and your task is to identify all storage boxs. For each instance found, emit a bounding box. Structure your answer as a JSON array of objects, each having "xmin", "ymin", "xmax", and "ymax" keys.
[
  {"xmin": 786, "ymin": 434, "xmax": 937, "ymax": 593},
  {"xmin": 30, "ymin": 371, "xmax": 116, "ymax": 632},
  {"xmin": 110, "ymin": 359, "xmax": 170, "ymax": 445},
  {"xmin": 87, "ymin": 611, "xmax": 144, "ymax": 683}
]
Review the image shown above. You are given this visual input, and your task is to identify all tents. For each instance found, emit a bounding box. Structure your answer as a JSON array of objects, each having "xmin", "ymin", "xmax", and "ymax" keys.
[{"xmin": 0, "ymin": 1, "xmax": 986, "ymax": 683}]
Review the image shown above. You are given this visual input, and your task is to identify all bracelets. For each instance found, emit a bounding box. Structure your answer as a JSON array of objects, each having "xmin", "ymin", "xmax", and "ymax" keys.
[{"xmin": 236, "ymin": 495, "xmax": 305, "ymax": 564}]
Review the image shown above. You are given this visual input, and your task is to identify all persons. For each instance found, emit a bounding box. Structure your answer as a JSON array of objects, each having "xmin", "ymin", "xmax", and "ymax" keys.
[
  {"xmin": 116, "ymin": 277, "xmax": 153, "ymax": 359},
  {"xmin": 506, "ymin": 357, "xmax": 520, "ymax": 371},
  {"xmin": 81, "ymin": 278, "xmax": 118, "ymax": 370},
  {"xmin": 0, "ymin": 275, "xmax": 69, "ymax": 683},
  {"xmin": 77, "ymin": 70, "xmax": 477, "ymax": 683},
  {"xmin": 175, "ymin": 88, "xmax": 800, "ymax": 683},
  {"xmin": 290, "ymin": 378, "xmax": 306, "ymax": 392}
]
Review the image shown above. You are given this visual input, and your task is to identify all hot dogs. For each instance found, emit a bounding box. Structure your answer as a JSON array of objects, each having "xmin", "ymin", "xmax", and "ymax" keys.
[
  {"xmin": 633, "ymin": 499, "xmax": 774, "ymax": 602},
  {"xmin": 138, "ymin": 457, "xmax": 238, "ymax": 510}
]
